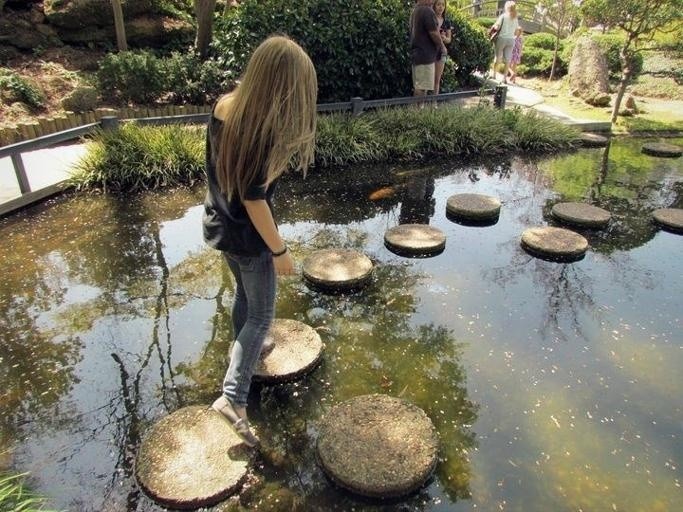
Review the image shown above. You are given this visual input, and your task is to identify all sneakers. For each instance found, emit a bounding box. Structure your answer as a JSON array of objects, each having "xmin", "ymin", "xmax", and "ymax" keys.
[
  {"xmin": 210, "ymin": 392, "xmax": 263, "ymax": 451},
  {"xmin": 261, "ymin": 336, "xmax": 275, "ymax": 354}
]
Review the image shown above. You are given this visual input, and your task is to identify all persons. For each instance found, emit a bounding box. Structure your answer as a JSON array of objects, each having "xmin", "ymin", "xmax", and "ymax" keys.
[
  {"xmin": 430, "ymin": 0, "xmax": 452, "ymax": 107},
  {"xmin": 410, "ymin": 0, "xmax": 447, "ymax": 108},
  {"xmin": 508, "ymin": 26, "xmax": 522, "ymax": 84},
  {"xmin": 202, "ymin": 36, "xmax": 317, "ymax": 450},
  {"xmin": 487, "ymin": 0, "xmax": 518, "ymax": 84}
]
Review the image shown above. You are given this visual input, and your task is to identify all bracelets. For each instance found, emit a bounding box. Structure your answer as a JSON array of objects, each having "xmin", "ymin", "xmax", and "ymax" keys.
[{"xmin": 271, "ymin": 246, "xmax": 287, "ymax": 257}]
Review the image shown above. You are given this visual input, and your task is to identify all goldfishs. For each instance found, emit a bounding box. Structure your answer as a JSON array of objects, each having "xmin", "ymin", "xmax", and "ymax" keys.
[{"xmin": 369, "ymin": 183, "xmax": 401, "ymax": 202}]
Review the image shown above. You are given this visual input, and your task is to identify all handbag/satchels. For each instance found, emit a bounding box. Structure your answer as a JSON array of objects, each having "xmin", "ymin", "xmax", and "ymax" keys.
[{"xmin": 487, "ymin": 25, "xmax": 501, "ymax": 44}]
